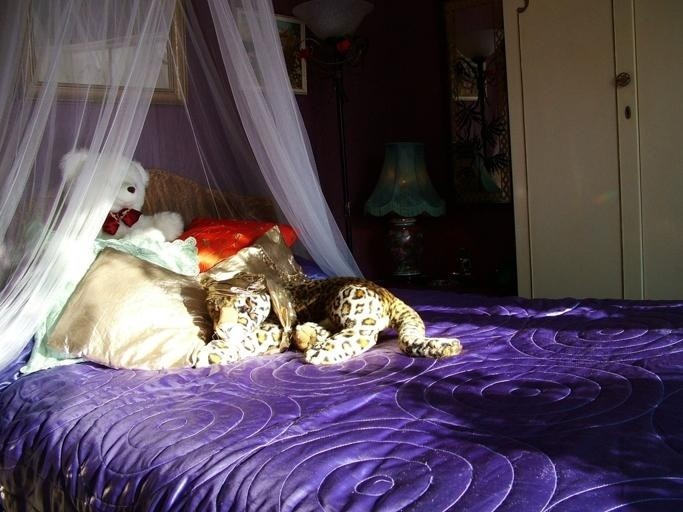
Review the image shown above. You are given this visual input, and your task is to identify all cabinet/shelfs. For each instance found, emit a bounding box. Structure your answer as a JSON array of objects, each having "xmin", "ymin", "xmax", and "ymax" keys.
[{"xmin": 501, "ymin": 0, "xmax": 683, "ymax": 300}]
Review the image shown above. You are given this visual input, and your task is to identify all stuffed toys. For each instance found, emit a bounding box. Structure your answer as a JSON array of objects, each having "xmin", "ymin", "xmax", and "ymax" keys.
[
  {"xmin": 60, "ymin": 149, "xmax": 201, "ymax": 275},
  {"xmin": 197, "ymin": 271, "xmax": 464, "ymax": 363}
]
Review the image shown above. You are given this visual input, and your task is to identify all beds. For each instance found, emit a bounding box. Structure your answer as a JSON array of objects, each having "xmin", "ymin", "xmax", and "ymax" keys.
[{"xmin": 0, "ymin": 171, "xmax": 682, "ymax": 510}]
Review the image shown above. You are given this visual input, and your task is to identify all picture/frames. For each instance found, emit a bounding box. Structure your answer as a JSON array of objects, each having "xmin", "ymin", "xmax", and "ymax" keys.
[
  {"xmin": 19, "ymin": 1, "xmax": 189, "ymax": 107},
  {"xmin": 273, "ymin": 13, "xmax": 307, "ymax": 96},
  {"xmin": 451, "ymin": 48, "xmax": 488, "ymax": 104}
]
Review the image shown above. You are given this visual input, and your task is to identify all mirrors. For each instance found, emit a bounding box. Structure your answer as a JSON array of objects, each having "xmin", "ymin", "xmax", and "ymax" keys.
[{"xmin": 439, "ymin": 1, "xmax": 512, "ymax": 208}]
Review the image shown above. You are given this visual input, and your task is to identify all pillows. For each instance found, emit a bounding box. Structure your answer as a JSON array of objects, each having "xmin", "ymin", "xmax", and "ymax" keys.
[{"xmin": 163, "ymin": 216, "xmax": 300, "ymax": 276}]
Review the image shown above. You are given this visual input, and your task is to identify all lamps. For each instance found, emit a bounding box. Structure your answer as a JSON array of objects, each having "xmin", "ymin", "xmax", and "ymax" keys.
[
  {"xmin": 290, "ymin": 1, "xmax": 375, "ymax": 254},
  {"xmin": 459, "ymin": 18, "xmax": 494, "ymax": 164},
  {"xmin": 364, "ymin": 141, "xmax": 447, "ymax": 277}
]
